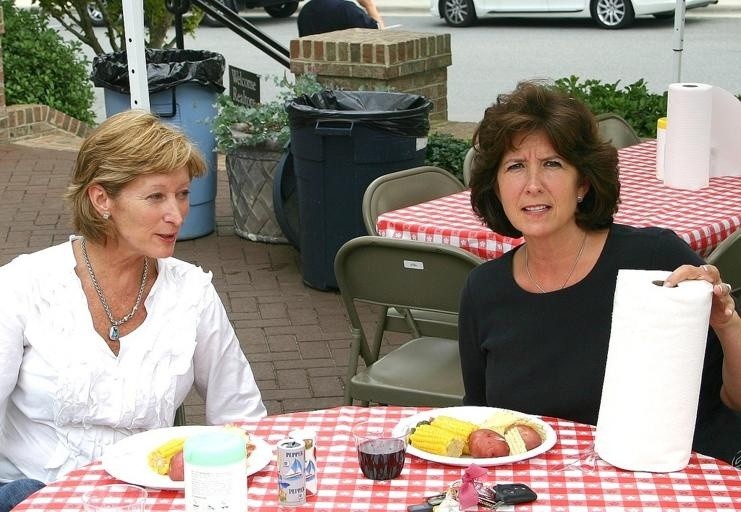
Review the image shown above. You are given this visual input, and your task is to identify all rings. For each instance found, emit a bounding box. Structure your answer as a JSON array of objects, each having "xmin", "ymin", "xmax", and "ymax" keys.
[{"xmin": 724, "ymin": 282, "xmax": 733, "ymax": 293}]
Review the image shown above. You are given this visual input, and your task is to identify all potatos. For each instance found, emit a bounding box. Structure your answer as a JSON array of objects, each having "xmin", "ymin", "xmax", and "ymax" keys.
[
  {"xmin": 469, "ymin": 429, "xmax": 511, "ymax": 458},
  {"xmin": 504, "ymin": 423, "xmax": 542, "ymax": 451},
  {"xmin": 168, "ymin": 450, "xmax": 184, "ymax": 481}
]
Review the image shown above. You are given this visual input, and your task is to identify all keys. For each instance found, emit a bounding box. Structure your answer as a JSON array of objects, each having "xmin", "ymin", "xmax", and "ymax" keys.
[{"xmin": 406, "ymin": 467, "xmax": 538, "ymax": 512}]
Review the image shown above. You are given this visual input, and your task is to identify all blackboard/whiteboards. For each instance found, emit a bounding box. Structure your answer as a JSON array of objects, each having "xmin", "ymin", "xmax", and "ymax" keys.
[{"xmin": 229, "ymin": 65, "xmax": 260, "ymax": 109}]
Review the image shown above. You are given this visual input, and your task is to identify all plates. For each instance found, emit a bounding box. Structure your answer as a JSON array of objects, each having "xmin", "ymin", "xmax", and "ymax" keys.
[
  {"xmin": 96, "ymin": 425, "xmax": 272, "ymax": 491},
  {"xmin": 391, "ymin": 404, "xmax": 557, "ymax": 466}
]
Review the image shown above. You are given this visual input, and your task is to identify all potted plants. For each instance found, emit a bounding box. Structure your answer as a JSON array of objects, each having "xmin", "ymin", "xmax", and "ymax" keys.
[{"xmin": 193, "ymin": 68, "xmax": 335, "ymax": 244}]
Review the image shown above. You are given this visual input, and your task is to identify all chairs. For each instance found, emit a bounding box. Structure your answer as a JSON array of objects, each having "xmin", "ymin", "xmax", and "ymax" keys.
[{"xmin": 175, "ymin": 258, "xmax": 199, "ymax": 425}]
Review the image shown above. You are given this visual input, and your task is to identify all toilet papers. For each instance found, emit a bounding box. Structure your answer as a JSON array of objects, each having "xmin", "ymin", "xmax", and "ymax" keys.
[
  {"xmin": 664, "ymin": 81, "xmax": 741, "ymax": 192},
  {"xmin": 594, "ymin": 264, "xmax": 713, "ymax": 472}
]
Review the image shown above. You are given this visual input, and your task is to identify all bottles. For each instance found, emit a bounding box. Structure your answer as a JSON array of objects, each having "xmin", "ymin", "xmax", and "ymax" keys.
[{"xmin": 182, "ymin": 430, "xmax": 249, "ymax": 512}]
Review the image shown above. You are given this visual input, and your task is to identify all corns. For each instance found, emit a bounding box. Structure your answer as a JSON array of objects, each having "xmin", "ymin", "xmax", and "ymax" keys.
[
  {"xmin": 409, "ymin": 424, "xmax": 465, "ymax": 457},
  {"xmin": 148, "ymin": 438, "xmax": 184, "ymax": 476},
  {"xmin": 430, "ymin": 415, "xmax": 480, "ymax": 455}
]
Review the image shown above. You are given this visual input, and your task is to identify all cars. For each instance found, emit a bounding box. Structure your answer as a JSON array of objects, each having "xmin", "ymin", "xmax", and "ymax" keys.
[
  {"xmin": 429, "ymin": 0, "xmax": 720, "ymax": 29},
  {"xmin": 76, "ymin": 1, "xmax": 299, "ymax": 26}
]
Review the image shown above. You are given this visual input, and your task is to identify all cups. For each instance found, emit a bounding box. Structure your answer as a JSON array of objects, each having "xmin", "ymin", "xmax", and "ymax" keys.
[
  {"xmin": 76, "ymin": 483, "xmax": 148, "ymax": 512},
  {"xmin": 351, "ymin": 418, "xmax": 411, "ymax": 482},
  {"xmin": 657, "ymin": 117, "xmax": 668, "ymax": 185}
]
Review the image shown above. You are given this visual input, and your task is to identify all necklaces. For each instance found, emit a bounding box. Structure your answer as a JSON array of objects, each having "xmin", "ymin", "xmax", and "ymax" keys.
[
  {"xmin": 525, "ymin": 228, "xmax": 588, "ymax": 295},
  {"xmin": 82, "ymin": 239, "xmax": 149, "ymax": 342}
]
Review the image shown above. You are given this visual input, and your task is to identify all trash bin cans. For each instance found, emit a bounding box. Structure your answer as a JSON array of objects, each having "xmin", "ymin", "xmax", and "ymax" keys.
[
  {"xmin": 93, "ymin": 48, "xmax": 226, "ymax": 241},
  {"xmin": 273, "ymin": 90, "xmax": 433, "ymax": 292}
]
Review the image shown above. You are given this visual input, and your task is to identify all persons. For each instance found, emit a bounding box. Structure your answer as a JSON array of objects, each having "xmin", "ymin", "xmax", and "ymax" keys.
[
  {"xmin": 0, "ymin": 109, "xmax": 269, "ymax": 511},
  {"xmin": 294, "ymin": 1, "xmax": 387, "ymax": 41},
  {"xmin": 456, "ymin": 79, "xmax": 741, "ymax": 466}
]
